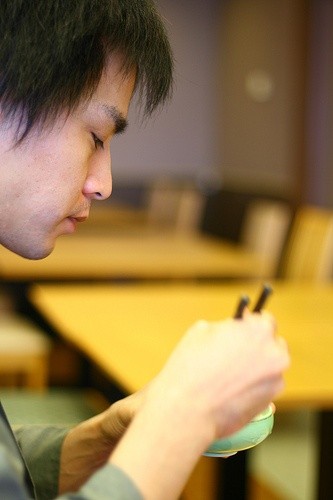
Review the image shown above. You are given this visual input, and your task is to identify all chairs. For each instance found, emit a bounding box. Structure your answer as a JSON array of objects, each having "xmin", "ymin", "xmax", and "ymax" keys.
[
  {"xmin": 147, "ymin": 189, "xmax": 332, "ymax": 280},
  {"xmin": 0, "ymin": 311, "xmax": 47, "ymax": 392}
]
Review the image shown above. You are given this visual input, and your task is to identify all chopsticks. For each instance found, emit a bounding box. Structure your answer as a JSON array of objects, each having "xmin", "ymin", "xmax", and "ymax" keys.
[{"xmin": 234, "ymin": 283, "xmax": 272, "ymax": 320}]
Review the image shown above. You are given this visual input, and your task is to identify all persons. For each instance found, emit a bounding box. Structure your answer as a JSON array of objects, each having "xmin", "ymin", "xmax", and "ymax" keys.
[{"xmin": 0, "ymin": 0, "xmax": 290, "ymax": 500}]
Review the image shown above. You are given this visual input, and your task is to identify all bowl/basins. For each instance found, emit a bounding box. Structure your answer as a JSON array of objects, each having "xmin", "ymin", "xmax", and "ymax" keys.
[{"xmin": 200, "ymin": 402, "xmax": 276, "ymax": 458}]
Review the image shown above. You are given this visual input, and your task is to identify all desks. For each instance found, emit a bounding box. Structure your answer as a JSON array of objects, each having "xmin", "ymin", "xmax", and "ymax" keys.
[
  {"xmin": 29, "ymin": 279, "xmax": 333, "ymax": 500},
  {"xmin": 0, "ymin": 203, "xmax": 260, "ymax": 280}
]
